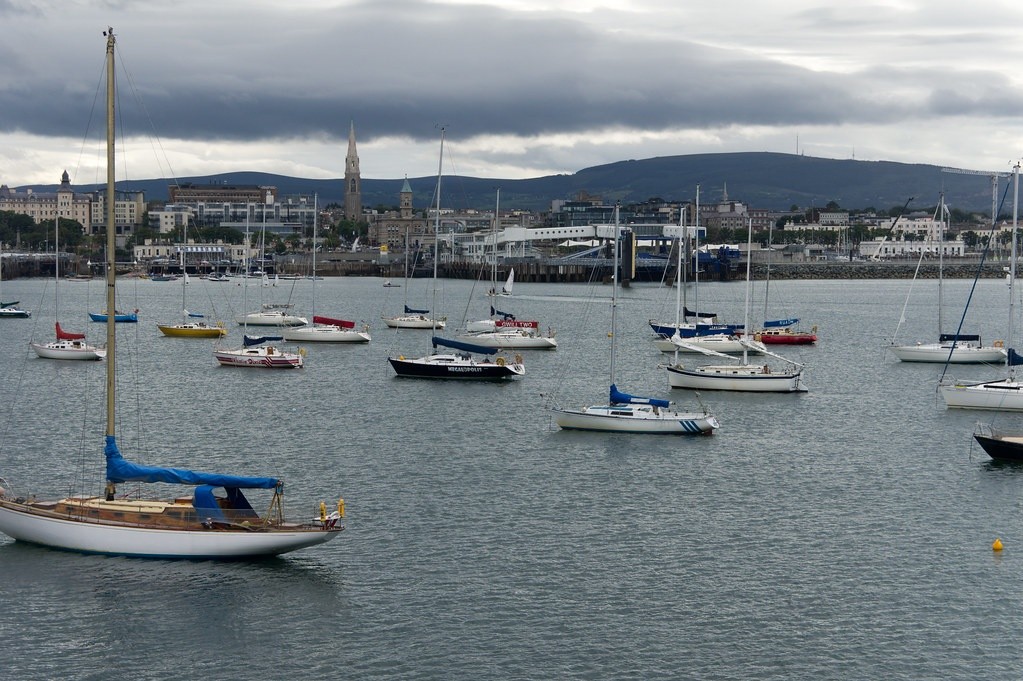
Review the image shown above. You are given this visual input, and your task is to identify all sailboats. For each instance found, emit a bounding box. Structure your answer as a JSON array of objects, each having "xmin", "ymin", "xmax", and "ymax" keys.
[
  {"xmin": 456, "ymin": 186, "xmax": 558, "ymax": 351},
  {"xmin": 380, "ymin": 226, "xmax": 446, "ymax": 329},
  {"xmin": 280, "ymin": 192, "xmax": 373, "ymax": 342},
  {"xmin": 386, "ymin": 127, "xmax": 526, "ymax": 379},
  {"xmin": 890, "ymin": 190, "xmax": 1009, "ymax": 364},
  {"xmin": 156, "ymin": 221, "xmax": 227, "ymax": 337},
  {"xmin": 546, "ymin": 198, "xmax": 720, "ymax": 434},
  {"xmin": 233, "ymin": 204, "xmax": 308, "ymax": 326},
  {"xmin": 0, "ymin": 25, "xmax": 346, "ymax": 562},
  {"xmin": 29, "ymin": 211, "xmax": 107, "ymax": 360},
  {"xmin": 935, "ymin": 159, "xmax": 1023, "ymax": 414},
  {"xmin": 647, "ymin": 185, "xmax": 818, "ymax": 393},
  {"xmin": 212, "ymin": 199, "xmax": 306, "ymax": 369},
  {"xmin": 502, "ymin": 267, "xmax": 514, "ymax": 295},
  {"xmin": 86, "ymin": 243, "xmax": 138, "ymax": 323}
]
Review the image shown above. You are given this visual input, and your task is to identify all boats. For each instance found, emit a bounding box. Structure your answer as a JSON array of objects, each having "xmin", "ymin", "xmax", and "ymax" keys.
[
  {"xmin": 198, "ymin": 271, "xmax": 230, "ymax": 281},
  {"xmin": 151, "ymin": 271, "xmax": 178, "ymax": 282},
  {"xmin": 971, "ymin": 419, "xmax": 1023, "ymax": 466},
  {"xmin": 382, "ymin": 281, "xmax": 401, "ymax": 287},
  {"xmin": 0, "ymin": 299, "xmax": 32, "ymax": 319}
]
